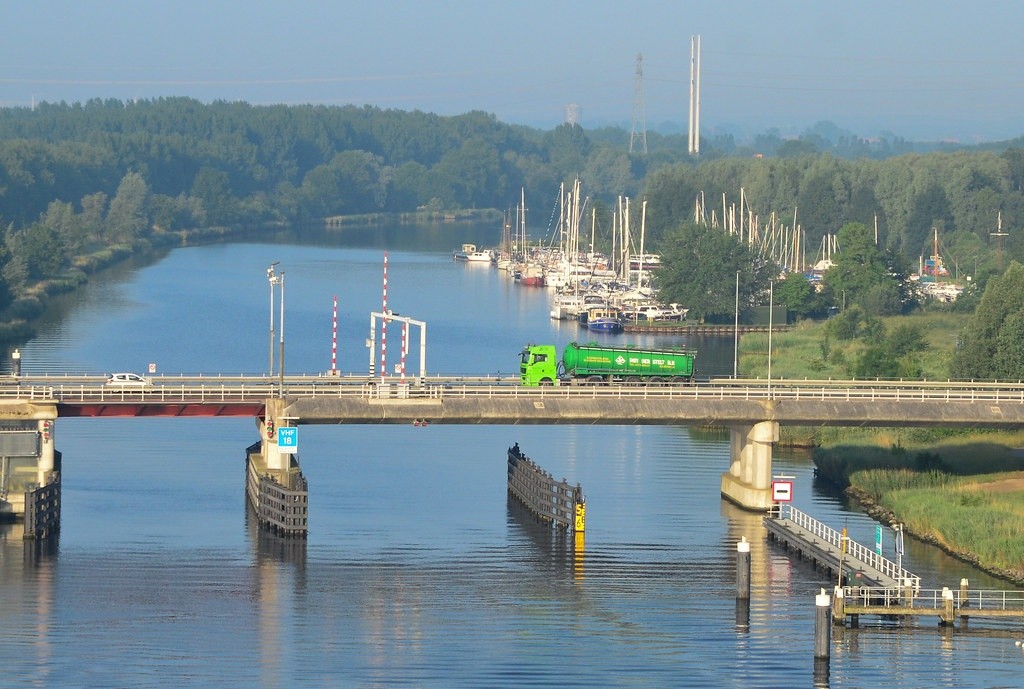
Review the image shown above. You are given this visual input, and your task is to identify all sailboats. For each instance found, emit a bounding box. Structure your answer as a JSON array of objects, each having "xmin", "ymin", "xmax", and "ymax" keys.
[
  {"xmin": 694, "ymin": 187, "xmax": 977, "ymax": 308},
  {"xmin": 454, "ymin": 177, "xmax": 690, "ymax": 333}
]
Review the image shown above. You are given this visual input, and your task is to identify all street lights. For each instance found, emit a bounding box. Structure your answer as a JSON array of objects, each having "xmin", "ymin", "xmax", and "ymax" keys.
[
  {"xmin": 734, "ymin": 267, "xmax": 742, "ymax": 379},
  {"xmin": 267, "ymin": 260, "xmax": 281, "ymax": 395}
]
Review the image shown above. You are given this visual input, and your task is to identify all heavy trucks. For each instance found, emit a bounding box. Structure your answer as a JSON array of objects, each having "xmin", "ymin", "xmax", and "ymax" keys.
[{"xmin": 518, "ymin": 340, "xmax": 698, "ymax": 388}]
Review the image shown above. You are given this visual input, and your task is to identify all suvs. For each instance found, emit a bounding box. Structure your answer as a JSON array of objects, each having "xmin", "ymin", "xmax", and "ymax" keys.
[{"xmin": 107, "ymin": 373, "xmax": 155, "ymax": 393}]
